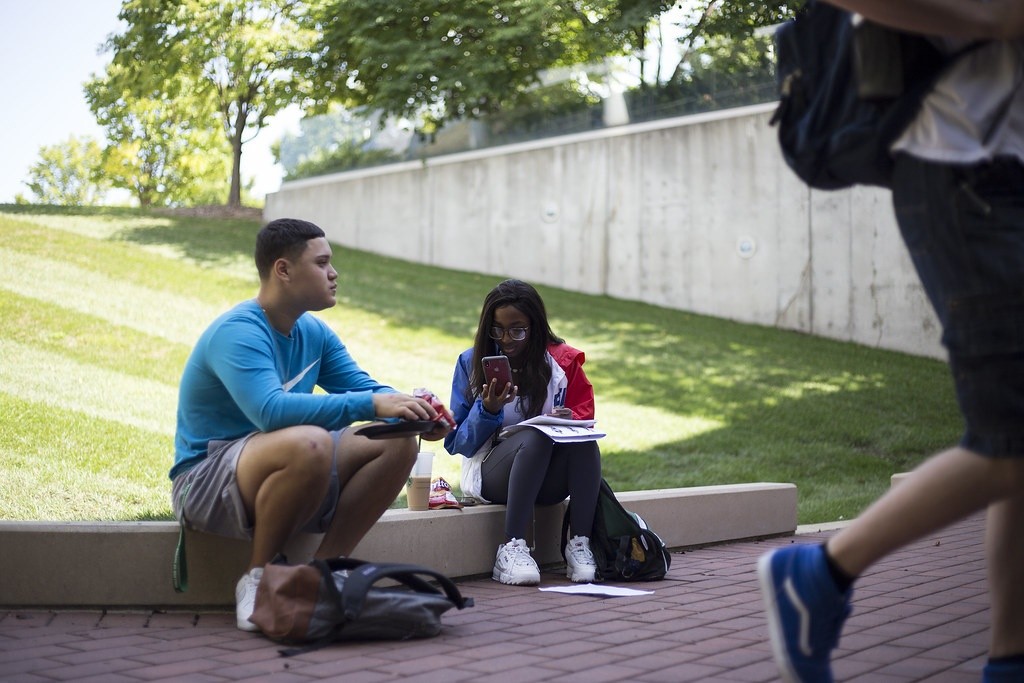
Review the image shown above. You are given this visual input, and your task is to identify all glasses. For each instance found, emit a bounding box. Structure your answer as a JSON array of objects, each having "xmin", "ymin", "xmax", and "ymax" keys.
[{"xmin": 488, "ymin": 326, "xmax": 529, "ymax": 340}]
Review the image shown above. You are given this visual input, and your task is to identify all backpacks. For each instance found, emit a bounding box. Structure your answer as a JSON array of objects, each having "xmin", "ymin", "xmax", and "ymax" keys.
[
  {"xmin": 246, "ymin": 557, "xmax": 475, "ymax": 656},
  {"xmin": 561, "ymin": 476, "xmax": 672, "ymax": 582},
  {"xmin": 769, "ymin": 2, "xmax": 1001, "ymax": 192}
]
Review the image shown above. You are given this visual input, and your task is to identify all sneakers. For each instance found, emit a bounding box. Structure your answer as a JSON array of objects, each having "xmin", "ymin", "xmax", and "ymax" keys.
[
  {"xmin": 234, "ymin": 567, "xmax": 263, "ymax": 631},
  {"xmin": 565, "ymin": 535, "xmax": 597, "ymax": 581},
  {"xmin": 758, "ymin": 543, "xmax": 853, "ymax": 683},
  {"xmin": 491, "ymin": 537, "xmax": 540, "ymax": 585}
]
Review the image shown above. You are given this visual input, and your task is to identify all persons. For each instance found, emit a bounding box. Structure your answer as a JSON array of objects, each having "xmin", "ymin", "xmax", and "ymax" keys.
[
  {"xmin": 441, "ymin": 279, "xmax": 604, "ymax": 586},
  {"xmin": 757, "ymin": 1, "xmax": 1024, "ymax": 683},
  {"xmin": 170, "ymin": 219, "xmax": 459, "ymax": 633}
]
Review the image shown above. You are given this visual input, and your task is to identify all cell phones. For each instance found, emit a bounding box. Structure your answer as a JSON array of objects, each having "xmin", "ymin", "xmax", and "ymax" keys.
[{"xmin": 482, "ymin": 355, "xmax": 514, "ymax": 397}]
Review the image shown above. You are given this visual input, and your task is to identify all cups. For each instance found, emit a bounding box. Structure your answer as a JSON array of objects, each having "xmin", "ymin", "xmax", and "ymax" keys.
[{"xmin": 405, "ymin": 452, "xmax": 435, "ymax": 511}]
